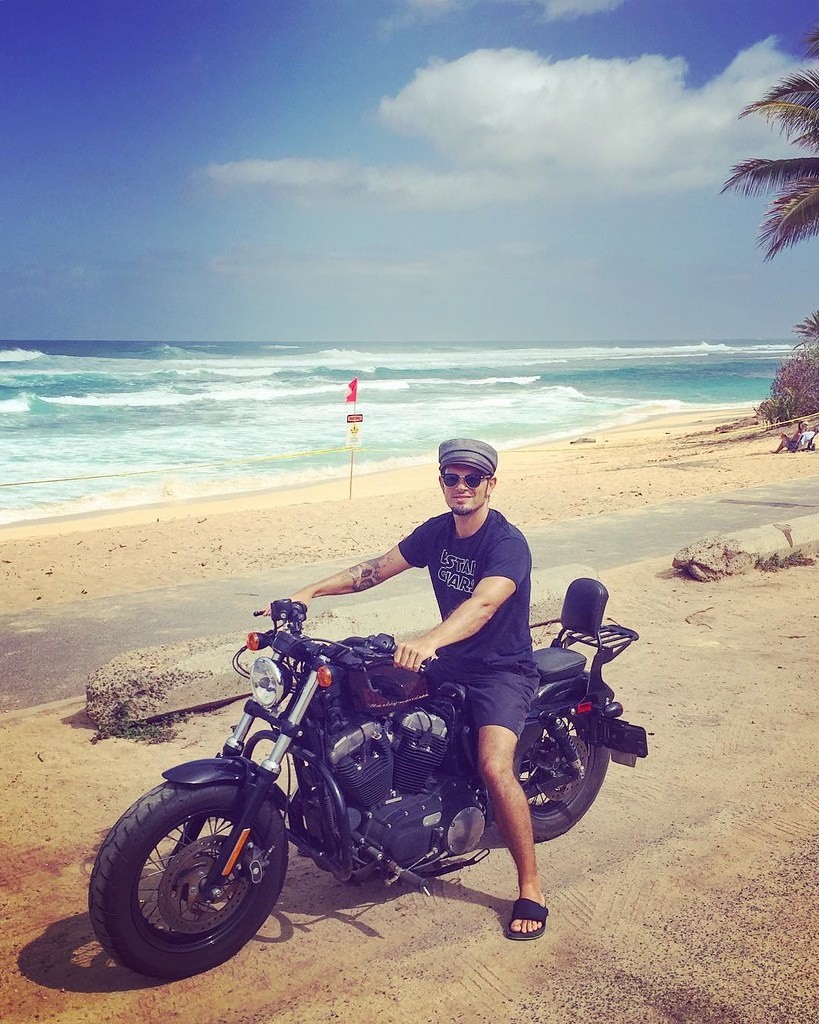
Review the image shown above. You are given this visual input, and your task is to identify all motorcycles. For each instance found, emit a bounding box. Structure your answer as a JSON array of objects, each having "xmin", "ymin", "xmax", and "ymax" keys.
[{"xmin": 84, "ymin": 573, "xmax": 649, "ymax": 982}]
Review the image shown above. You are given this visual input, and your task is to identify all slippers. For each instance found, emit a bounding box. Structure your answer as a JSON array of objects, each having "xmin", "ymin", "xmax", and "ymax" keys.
[{"xmin": 506, "ymin": 898, "xmax": 548, "ymax": 939}]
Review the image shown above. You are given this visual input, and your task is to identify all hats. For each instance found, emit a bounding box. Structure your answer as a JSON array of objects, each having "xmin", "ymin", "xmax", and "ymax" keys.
[{"xmin": 438, "ymin": 438, "xmax": 498, "ymax": 475}]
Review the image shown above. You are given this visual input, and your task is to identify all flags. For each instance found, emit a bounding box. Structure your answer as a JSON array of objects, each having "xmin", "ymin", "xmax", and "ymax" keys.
[{"xmin": 346, "ymin": 377, "xmax": 357, "ymax": 403}]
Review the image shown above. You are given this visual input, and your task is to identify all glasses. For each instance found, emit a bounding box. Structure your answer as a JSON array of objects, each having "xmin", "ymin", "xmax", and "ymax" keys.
[{"xmin": 441, "ymin": 473, "xmax": 490, "ymax": 489}]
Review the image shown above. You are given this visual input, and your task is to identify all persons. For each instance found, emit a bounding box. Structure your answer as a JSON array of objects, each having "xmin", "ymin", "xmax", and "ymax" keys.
[
  {"xmin": 263, "ymin": 438, "xmax": 549, "ymax": 941},
  {"xmin": 770, "ymin": 420, "xmax": 809, "ymax": 454}
]
